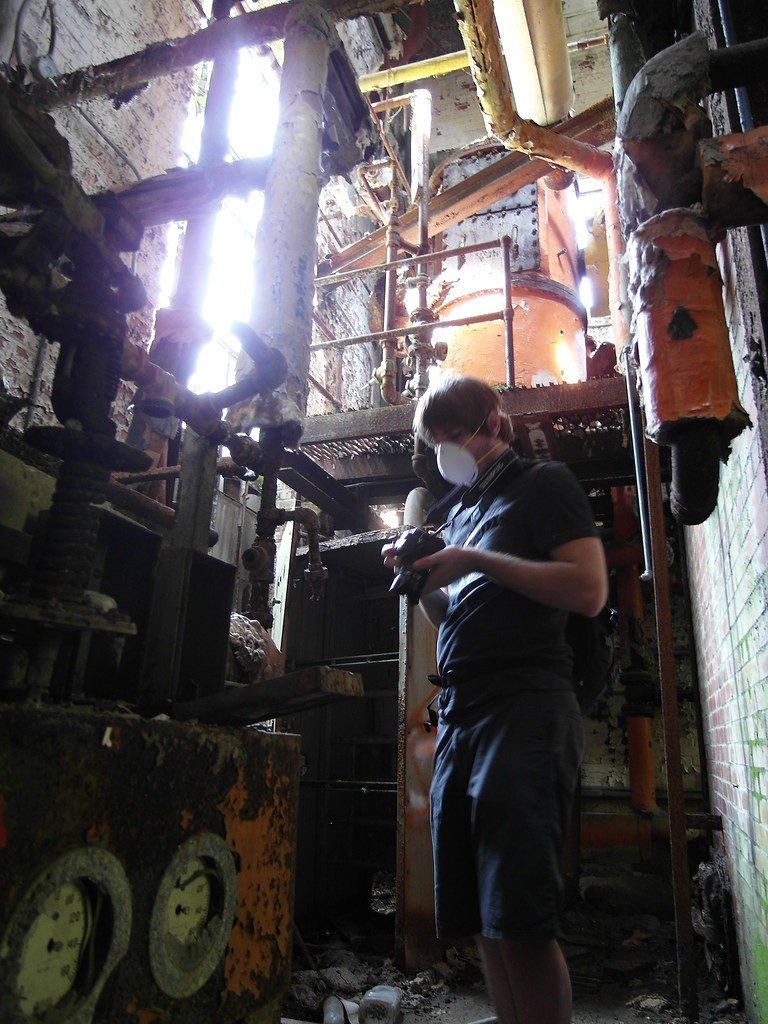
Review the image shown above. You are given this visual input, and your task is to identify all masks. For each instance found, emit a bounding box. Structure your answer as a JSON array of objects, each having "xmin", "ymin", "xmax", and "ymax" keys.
[{"xmin": 434, "ymin": 417, "xmax": 502, "ymax": 487}]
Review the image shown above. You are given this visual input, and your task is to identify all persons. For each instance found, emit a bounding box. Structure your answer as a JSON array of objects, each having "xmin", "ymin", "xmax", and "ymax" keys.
[{"xmin": 381, "ymin": 377, "xmax": 610, "ymax": 1023}]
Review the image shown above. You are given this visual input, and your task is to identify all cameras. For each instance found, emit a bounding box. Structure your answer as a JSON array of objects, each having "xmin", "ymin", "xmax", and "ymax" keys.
[{"xmin": 388, "ymin": 526, "xmax": 447, "ymax": 610}]
